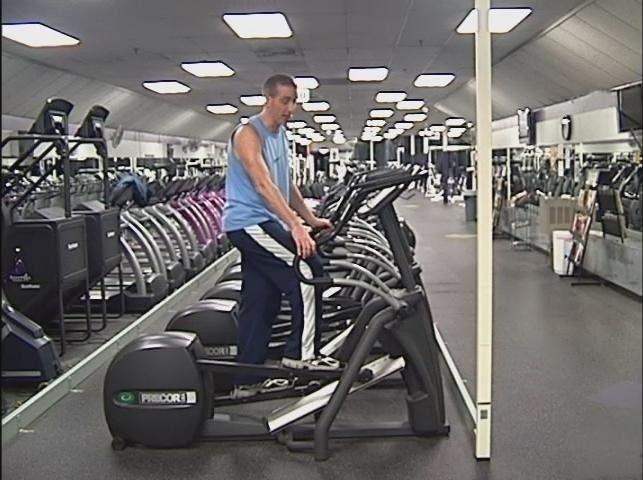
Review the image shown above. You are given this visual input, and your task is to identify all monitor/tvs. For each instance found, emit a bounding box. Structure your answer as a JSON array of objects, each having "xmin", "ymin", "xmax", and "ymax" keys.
[
  {"xmin": 78, "ymin": 105, "xmax": 109, "ymax": 138},
  {"xmin": 34, "ymin": 99, "xmax": 73, "ymax": 136},
  {"xmin": 618, "ymin": 83, "xmax": 643, "ymax": 132},
  {"xmin": 518, "ymin": 108, "xmax": 531, "ymax": 143}
]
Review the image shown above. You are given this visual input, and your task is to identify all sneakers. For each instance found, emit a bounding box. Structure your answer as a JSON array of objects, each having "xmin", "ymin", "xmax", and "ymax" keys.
[
  {"xmin": 229, "ymin": 376, "xmax": 296, "ymax": 400},
  {"xmin": 280, "ymin": 355, "xmax": 350, "ymax": 373}
]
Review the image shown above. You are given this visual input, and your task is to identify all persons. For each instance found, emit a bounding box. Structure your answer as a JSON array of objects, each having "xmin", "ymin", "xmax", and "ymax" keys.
[{"xmin": 222, "ymin": 75, "xmax": 336, "ymax": 400}]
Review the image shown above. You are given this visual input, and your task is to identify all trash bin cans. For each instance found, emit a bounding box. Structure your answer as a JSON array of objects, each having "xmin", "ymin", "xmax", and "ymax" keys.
[
  {"xmin": 552, "ymin": 230, "xmax": 575, "ymax": 276},
  {"xmin": 464, "ymin": 195, "xmax": 476, "ymax": 220}
]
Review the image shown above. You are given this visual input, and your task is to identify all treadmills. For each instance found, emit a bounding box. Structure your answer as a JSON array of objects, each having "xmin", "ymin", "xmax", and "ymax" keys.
[{"xmin": 66, "ymin": 171, "xmax": 234, "ymax": 314}]
